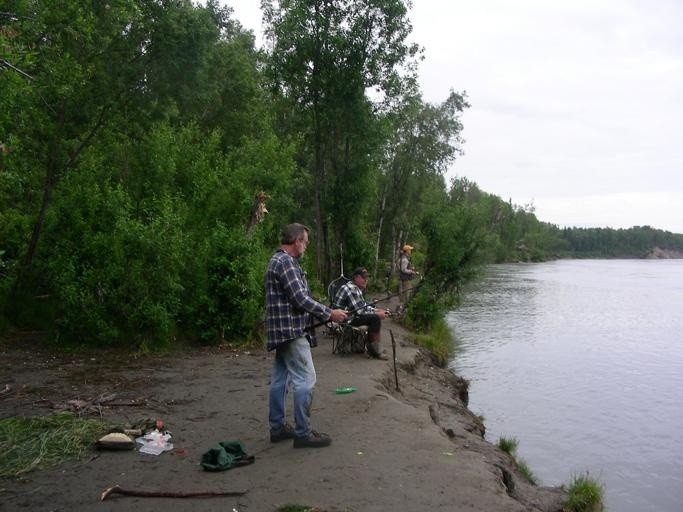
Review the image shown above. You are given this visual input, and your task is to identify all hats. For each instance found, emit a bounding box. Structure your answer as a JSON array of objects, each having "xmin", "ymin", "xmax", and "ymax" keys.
[
  {"xmin": 403, "ymin": 245, "xmax": 414, "ymax": 251},
  {"xmin": 352, "ymin": 267, "xmax": 372, "ymax": 276}
]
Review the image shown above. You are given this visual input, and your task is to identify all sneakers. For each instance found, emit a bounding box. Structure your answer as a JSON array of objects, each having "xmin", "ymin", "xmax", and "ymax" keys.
[
  {"xmin": 293, "ymin": 429, "xmax": 331, "ymax": 448},
  {"xmin": 270, "ymin": 422, "xmax": 295, "ymax": 442}
]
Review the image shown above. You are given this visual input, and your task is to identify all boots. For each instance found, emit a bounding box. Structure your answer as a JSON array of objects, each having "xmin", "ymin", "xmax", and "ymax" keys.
[{"xmin": 366, "ymin": 339, "xmax": 391, "ymax": 360}]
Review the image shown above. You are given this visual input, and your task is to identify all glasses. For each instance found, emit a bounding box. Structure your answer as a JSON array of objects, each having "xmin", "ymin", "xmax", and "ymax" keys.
[{"xmin": 301, "ymin": 239, "xmax": 310, "ymax": 247}]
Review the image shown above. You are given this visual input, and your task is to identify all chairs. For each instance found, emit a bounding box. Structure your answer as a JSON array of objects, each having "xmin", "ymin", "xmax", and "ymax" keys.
[{"xmin": 328, "ymin": 274, "xmax": 376, "ymax": 354}]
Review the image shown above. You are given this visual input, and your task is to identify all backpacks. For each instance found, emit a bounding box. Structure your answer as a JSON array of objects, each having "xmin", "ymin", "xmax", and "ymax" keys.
[{"xmin": 394, "ymin": 258, "xmax": 401, "ymax": 274}]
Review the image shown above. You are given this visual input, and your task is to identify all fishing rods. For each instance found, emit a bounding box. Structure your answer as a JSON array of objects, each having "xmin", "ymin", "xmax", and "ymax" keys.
[
  {"xmin": 305, "ymin": 267, "xmax": 457, "ymax": 333},
  {"xmin": 369, "ymin": 306, "xmax": 482, "ymax": 317}
]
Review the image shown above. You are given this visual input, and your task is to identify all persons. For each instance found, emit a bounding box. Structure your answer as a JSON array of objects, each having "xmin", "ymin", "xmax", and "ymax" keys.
[
  {"xmin": 332, "ymin": 267, "xmax": 391, "ymax": 361},
  {"xmin": 263, "ymin": 222, "xmax": 348, "ymax": 447},
  {"xmin": 395, "ymin": 245, "xmax": 419, "ymax": 280}
]
